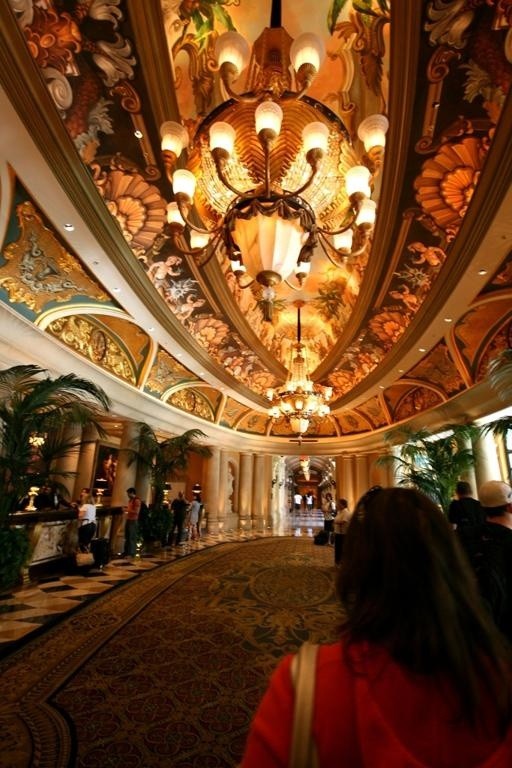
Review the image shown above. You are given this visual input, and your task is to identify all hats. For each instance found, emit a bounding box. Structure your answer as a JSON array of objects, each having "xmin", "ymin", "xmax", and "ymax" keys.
[{"xmin": 479, "ymin": 480, "xmax": 512, "ymax": 508}]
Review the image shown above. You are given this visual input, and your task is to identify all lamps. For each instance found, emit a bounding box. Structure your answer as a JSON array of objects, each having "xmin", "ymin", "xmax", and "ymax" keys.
[
  {"xmin": 264, "ymin": 295, "xmax": 334, "ymax": 441},
  {"xmin": 159, "ymin": 1, "xmax": 388, "ymax": 324}
]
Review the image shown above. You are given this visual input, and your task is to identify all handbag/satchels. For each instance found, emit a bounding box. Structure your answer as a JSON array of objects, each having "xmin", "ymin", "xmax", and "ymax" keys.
[
  {"xmin": 76, "ymin": 553, "xmax": 94, "ymax": 567},
  {"xmin": 315, "ymin": 530, "xmax": 329, "ymax": 545}
]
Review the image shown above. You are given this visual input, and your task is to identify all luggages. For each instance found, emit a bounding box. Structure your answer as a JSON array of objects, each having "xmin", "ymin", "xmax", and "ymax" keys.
[{"xmin": 90, "ymin": 537, "xmax": 110, "ymax": 568}]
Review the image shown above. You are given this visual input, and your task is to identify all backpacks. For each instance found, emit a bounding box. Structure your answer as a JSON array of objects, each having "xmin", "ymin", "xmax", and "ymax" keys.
[{"xmin": 132, "ymin": 497, "xmax": 148, "ymax": 523}]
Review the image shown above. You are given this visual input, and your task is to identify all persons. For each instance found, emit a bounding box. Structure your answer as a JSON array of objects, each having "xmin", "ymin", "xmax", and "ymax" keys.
[
  {"xmin": 386, "ymin": 285, "xmax": 426, "ymax": 313},
  {"xmin": 306, "ymin": 491, "xmax": 314, "ymax": 517},
  {"xmin": 146, "ymin": 254, "xmax": 182, "ymax": 293},
  {"xmin": 333, "ymin": 497, "xmax": 355, "ymax": 564},
  {"xmin": 235, "ymin": 484, "xmax": 512, "ymax": 765},
  {"xmin": 292, "ymin": 490, "xmax": 303, "ymax": 515},
  {"xmin": 319, "ymin": 490, "xmax": 335, "ymax": 549},
  {"xmin": 169, "ymin": 491, "xmax": 187, "ymax": 547},
  {"xmin": 78, "ymin": 495, "xmax": 98, "ymax": 554},
  {"xmin": 119, "ymin": 487, "xmax": 142, "ymax": 559},
  {"xmin": 448, "ymin": 480, "xmax": 482, "ymax": 528},
  {"xmin": 406, "ymin": 238, "xmax": 445, "ymax": 274},
  {"xmin": 49, "ymin": 487, "xmax": 78, "ymax": 510},
  {"xmin": 81, "ymin": 488, "xmax": 91, "ymax": 504},
  {"xmin": 189, "ymin": 494, "xmax": 202, "ymax": 541},
  {"xmin": 173, "ymin": 294, "xmax": 206, "ymax": 321},
  {"xmin": 458, "ymin": 478, "xmax": 512, "ymax": 648}
]
